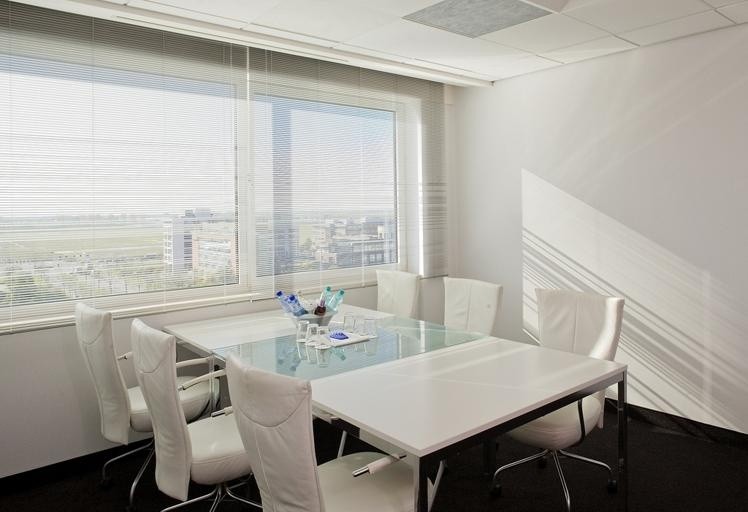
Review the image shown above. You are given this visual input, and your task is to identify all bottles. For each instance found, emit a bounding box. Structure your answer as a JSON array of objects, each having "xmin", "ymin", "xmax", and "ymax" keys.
[
  {"xmin": 313, "ymin": 286, "xmax": 345, "ymax": 316},
  {"xmin": 276, "ymin": 291, "xmax": 307, "ymax": 316}
]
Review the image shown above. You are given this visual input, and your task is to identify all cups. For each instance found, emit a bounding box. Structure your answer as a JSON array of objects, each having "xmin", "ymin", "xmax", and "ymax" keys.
[
  {"xmin": 348, "ymin": 337, "xmax": 377, "ymax": 355},
  {"xmin": 296, "ymin": 320, "xmax": 331, "ymax": 349},
  {"xmin": 297, "ymin": 341, "xmax": 331, "ymax": 367},
  {"xmin": 342, "ymin": 315, "xmax": 377, "ymax": 337}
]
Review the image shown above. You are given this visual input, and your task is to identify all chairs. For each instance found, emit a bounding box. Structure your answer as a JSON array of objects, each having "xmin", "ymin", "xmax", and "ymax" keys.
[
  {"xmin": 76, "ymin": 301, "xmax": 219, "ymax": 503},
  {"xmin": 130, "ymin": 318, "xmax": 264, "ymax": 512},
  {"xmin": 224, "ymin": 351, "xmax": 444, "ymax": 512},
  {"xmin": 443, "ymin": 275, "xmax": 504, "ymax": 336},
  {"xmin": 372, "ymin": 272, "xmax": 420, "ymax": 317}
]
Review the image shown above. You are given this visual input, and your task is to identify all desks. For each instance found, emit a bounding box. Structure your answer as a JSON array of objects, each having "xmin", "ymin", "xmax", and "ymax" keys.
[
  {"xmin": 161, "ymin": 296, "xmax": 625, "ymax": 512},
  {"xmin": 491, "ymin": 287, "xmax": 625, "ymax": 509}
]
